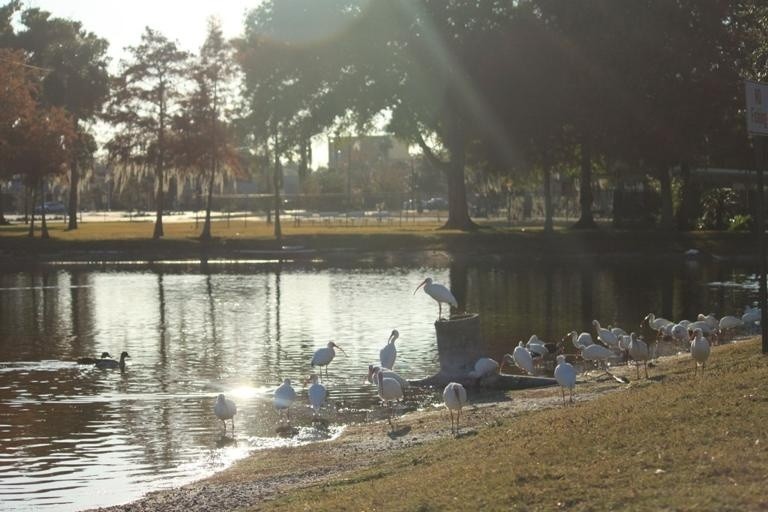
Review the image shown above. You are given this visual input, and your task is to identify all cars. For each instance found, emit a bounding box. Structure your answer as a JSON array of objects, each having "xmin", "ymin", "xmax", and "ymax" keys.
[
  {"xmin": 35, "ymin": 201, "xmax": 67, "ymax": 213},
  {"xmin": 404, "ymin": 197, "xmax": 449, "ymax": 211}
]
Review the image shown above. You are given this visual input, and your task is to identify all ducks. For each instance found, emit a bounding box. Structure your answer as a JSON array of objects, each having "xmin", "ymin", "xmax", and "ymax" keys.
[{"xmin": 76, "ymin": 351, "xmax": 130, "ymax": 370}]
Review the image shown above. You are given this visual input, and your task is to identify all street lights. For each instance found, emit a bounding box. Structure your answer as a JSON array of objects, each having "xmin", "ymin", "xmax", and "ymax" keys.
[{"xmin": 346, "ymin": 140, "xmax": 361, "ymax": 217}]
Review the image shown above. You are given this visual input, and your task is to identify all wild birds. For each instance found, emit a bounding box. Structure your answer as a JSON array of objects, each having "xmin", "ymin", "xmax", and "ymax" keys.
[
  {"xmin": 214, "ymin": 394, "xmax": 237, "ymax": 436},
  {"xmin": 467, "ymin": 301, "xmax": 762, "ymax": 406},
  {"xmin": 442, "ymin": 382, "xmax": 467, "ymax": 433},
  {"xmin": 414, "ymin": 278, "xmax": 459, "ymax": 320},
  {"xmin": 274, "ymin": 342, "xmax": 348, "ymax": 427},
  {"xmin": 368, "ymin": 330, "xmax": 406, "ymax": 424}
]
